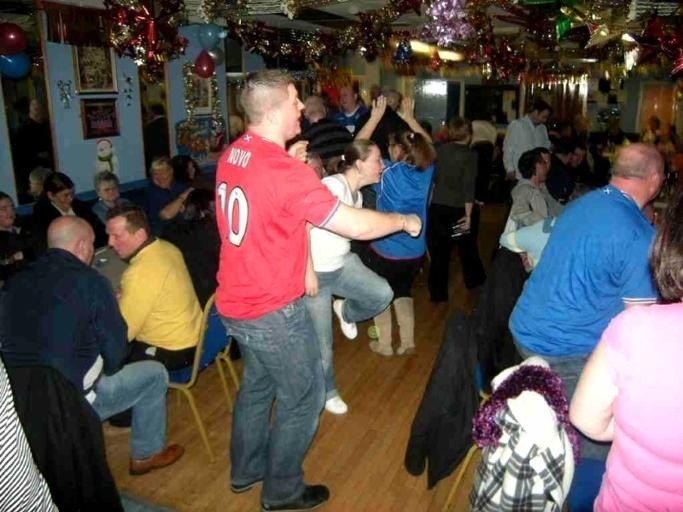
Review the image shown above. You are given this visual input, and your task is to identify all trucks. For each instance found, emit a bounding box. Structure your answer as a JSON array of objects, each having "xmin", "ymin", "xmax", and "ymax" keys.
[
  {"xmin": 0, "ymin": 22, "xmax": 28, "ymax": 56},
  {"xmin": 0, "ymin": 53, "xmax": 31, "ymax": 78},
  {"xmin": 195, "ymin": 50, "xmax": 214, "ymax": 79},
  {"xmin": 206, "ymin": 49, "xmax": 226, "ymax": 66},
  {"xmin": 199, "ymin": 22, "xmax": 220, "ymax": 50}
]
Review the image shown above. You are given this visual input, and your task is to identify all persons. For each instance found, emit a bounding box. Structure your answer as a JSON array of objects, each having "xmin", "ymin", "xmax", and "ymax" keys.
[
  {"xmin": 32, "ymin": 172, "xmax": 109, "ymax": 254},
  {"xmin": 502, "ymin": 100, "xmax": 552, "ymax": 207},
  {"xmin": 11, "ymin": 97, "xmax": 55, "ymax": 204},
  {"xmin": 331, "ymin": 82, "xmax": 366, "ymax": 134},
  {"xmin": 0, "ymin": 361, "xmax": 58, "ymax": 512},
  {"xmin": 287, "ymin": 95, "xmax": 353, "ymax": 176},
  {"xmin": 302, "ymin": 139, "xmax": 393, "ymax": 414},
  {"xmin": 642, "ymin": 116, "xmax": 683, "ymax": 173},
  {"xmin": 169, "ymin": 155, "xmax": 222, "ymax": 247},
  {"xmin": 104, "ymin": 202, "xmax": 204, "ymax": 428},
  {"xmin": 425, "ymin": 117, "xmax": 488, "ymax": 305},
  {"xmin": 214, "ymin": 70, "xmax": 422, "ymax": 510},
  {"xmin": 507, "ymin": 143, "xmax": 661, "ymax": 462},
  {"xmin": 84, "ymin": 170, "xmax": 134, "ymax": 246},
  {"xmin": 469, "ymin": 120, "xmax": 497, "ymax": 206},
  {"xmin": 144, "ymin": 155, "xmax": 203, "ymax": 262},
  {"xmin": 0, "ymin": 192, "xmax": 36, "ymax": 266},
  {"xmin": 568, "ymin": 197, "xmax": 683, "ymax": 511},
  {"xmin": 354, "ymin": 90, "xmax": 409, "ymax": 158},
  {"xmin": 355, "ymin": 95, "xmax": 437, "ymax": 355},
  {"xmin": 509, "ymin": 143, "xmax": 592, "ymax": 225}
]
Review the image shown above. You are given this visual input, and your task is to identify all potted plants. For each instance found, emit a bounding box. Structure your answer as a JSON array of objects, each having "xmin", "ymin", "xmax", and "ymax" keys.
[
  {"xmin": 82, "ymin": 98, "xmax": 120, "ymax": 137},
  {"xmin": 188, "ymin": 71, "xmax": 212, "ymax": 115},
  {"xmin": 72, "ymin": 45, "xmax": 119, "ymax": 95}
]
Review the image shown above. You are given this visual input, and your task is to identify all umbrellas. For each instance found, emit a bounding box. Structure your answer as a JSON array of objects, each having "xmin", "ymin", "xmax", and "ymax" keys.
[
  {"xmin": 262, "ymin": 485, "xmax": 332, "ymax": 512},
  {"xmin": 129, "ymin": 443, "xmax": 185, "ymax": 477},
  {"xmin": 231, "ymin": 478, "xmax": 265, "ymax": 493},
  {"xmin": 431, "ymin": 300, "xmax": 449, "ymax": 312}
]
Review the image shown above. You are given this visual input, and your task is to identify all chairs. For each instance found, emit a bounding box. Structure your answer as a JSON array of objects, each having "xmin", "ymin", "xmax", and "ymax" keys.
[
  {"xmin": 326, "ymin": 395, "xmax": 349, "ymax": 415},
  {"xmin": 474, "ymin": 200, "xmax": 484, "ymax": 206},
  {"xmin": 334, "ymin": 299, "xmax": 359, "ymax": 340}
]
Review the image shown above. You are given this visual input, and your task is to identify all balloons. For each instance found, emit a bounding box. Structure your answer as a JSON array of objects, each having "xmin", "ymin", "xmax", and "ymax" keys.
[
  {"xmin": 199, "ymin": 22, "xmax": 220, "ymax": 50},
  {"xmin": 0, "ymin": 22, "xmax": 28, "ymax": 56},
  {"xmin": 0, "ymin": 53, "xmax": 31, "ymax": 78},
  {"xmin": 195, "ymin": 50, "xmax": 214, "ymax": 79},
  {"xmin": 206, "ymin": 49, "xmax": 226, "ymax": 66}
]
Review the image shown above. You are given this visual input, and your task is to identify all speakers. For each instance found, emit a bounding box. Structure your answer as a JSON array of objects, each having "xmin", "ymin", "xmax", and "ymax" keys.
[
  {"xmin": 394, "ymin": 296, "xmax": 419, "ymax": 358},
  {"xmin": 369, "ymin": 305, "xmax": 396, "ymax": 358}
]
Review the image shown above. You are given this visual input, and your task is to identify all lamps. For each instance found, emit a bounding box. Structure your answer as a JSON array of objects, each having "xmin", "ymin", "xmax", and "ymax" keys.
[
  {"xmin": 435, "ymin": 390, "xmax": 607, "ymax": 512},
  {"xmin": 163, "ymin": 294, "xmax": 242, "ymax": 464}
]
